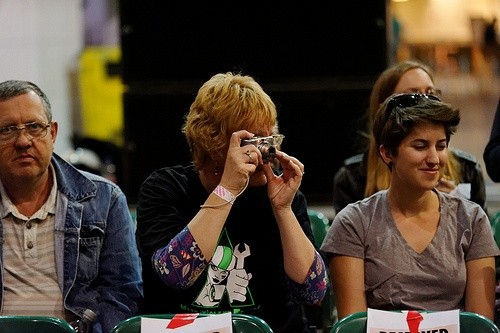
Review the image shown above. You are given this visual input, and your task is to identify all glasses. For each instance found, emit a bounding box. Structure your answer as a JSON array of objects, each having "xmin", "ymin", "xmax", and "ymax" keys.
[
  {"xmin": 384, "ymin": 95, "xmax": 447, "ymax": 119},
  {"xmin": 396, "ymin": 89, "xmax": 444, "ymax": 96},
  {"xmin": 0, "ymin": 121, "xmax": 50, "ymax": 139}
]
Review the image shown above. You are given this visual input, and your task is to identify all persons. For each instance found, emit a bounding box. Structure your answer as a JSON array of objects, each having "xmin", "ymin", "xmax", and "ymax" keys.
[
  {"xmin": 320, "ymin": 93, "xmax": 500, "ymax": 324},
  {"xmin": 334, "ymin": 62, "xmax": 487, "ymax": 216},
  {"xmin": 0, "ymin": 81, "xmax": 144, "ymax": 333},
  {"xmin": 482, "ymin": 98, "xmax": 500, "ymax": 183},
  {"xmin": 134, "ymin": 73, "xmax": 333, "ymax": 333}
]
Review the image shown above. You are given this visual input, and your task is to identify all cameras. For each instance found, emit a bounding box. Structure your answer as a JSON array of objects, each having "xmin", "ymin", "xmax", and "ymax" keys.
[{"xmin": 243, "ymin": 136, "xmax": 276, "ymax": 160}]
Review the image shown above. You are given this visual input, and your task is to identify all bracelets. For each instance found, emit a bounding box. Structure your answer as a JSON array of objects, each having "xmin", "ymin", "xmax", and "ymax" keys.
[{"xmin": 212, "ymin": 184, "xmax": 236, "ymax": 204}]
